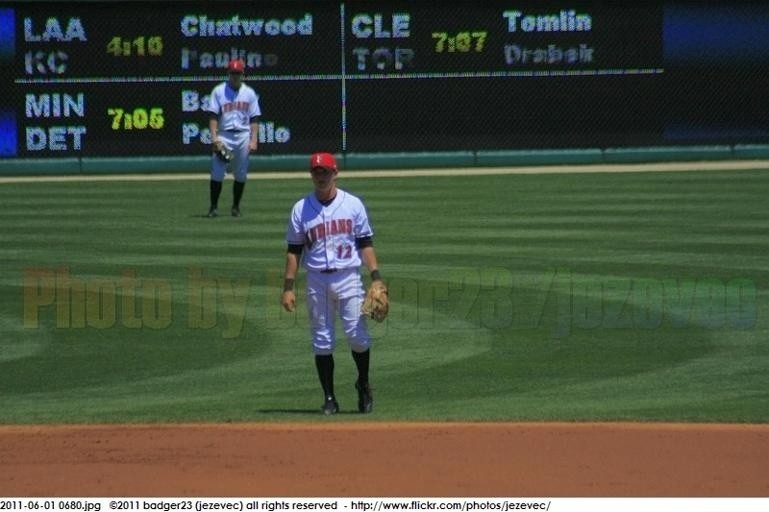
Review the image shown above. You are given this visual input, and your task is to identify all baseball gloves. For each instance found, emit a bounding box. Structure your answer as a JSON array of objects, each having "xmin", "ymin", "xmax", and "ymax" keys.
[
  {"xmin": 214, "ymin": 141, "xmax": 234, "ymax": 162},
  {"xmin": 362, "ymin": 281, "xmax": 388, "ymax": 322}
]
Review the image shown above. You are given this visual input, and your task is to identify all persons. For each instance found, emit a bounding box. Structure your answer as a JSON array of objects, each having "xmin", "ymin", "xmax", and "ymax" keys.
[
  {"xmin": 204, "ymin": 59, "xmax": 262, "ymax": 218},
  {"xmin": 281, "ymin": 150, "xmax": 390, "ymax": 416}
]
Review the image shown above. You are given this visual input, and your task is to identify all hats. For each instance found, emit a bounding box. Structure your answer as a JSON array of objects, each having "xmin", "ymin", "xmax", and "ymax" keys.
[
  {"xmin": 226, "ymin": 60, "xmax": 246, "ymax": 74},
  {"xmin": 309, "ymin": 153, "xmax": 339, "ymax": 172}
]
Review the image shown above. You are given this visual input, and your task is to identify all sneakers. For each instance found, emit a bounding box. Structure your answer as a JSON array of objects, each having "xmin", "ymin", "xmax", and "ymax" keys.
[
  {"xmin": 322, "ymin": 396, "xmax": 339, "ymax": 415},
  {"xmin": 208, "ymin": 209, "xmax": 218, "ymax": 217},
  {"xmin": 355, "ymin": 378, "xmax": 373, "ymax": 413},
  {"xmin": 231, "ymin": 207, "xmax": 241, "ymax": 216}
]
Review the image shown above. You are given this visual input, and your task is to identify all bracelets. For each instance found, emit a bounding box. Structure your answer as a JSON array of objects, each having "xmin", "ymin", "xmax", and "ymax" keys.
[
  {"xmin": 369, "ymin": 269, "xmax": 382, "ymax": 282},
  {"xmin": 283, "ymin": 278, "xmax": 295, "ymax": 292}
]
